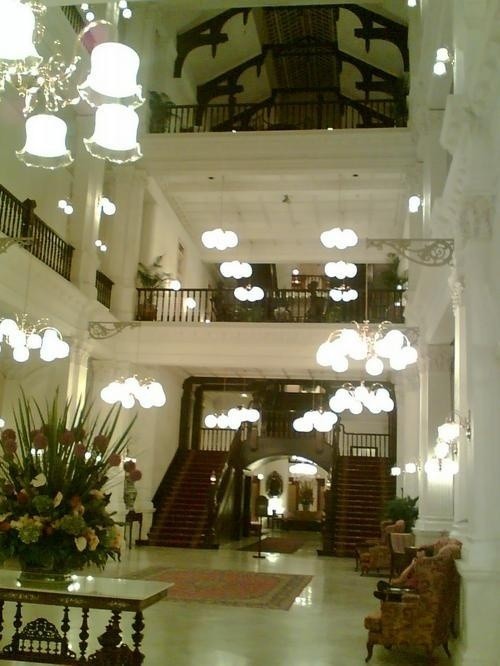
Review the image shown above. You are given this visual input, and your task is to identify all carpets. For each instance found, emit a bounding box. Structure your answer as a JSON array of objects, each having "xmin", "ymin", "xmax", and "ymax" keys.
[
  {"xmin": 237, "ymin": 537, "xmax": 306, "ymax": 554},
  {"xmin": 116, "ymin": 565, "xmax": 314, "ymax": 611}
]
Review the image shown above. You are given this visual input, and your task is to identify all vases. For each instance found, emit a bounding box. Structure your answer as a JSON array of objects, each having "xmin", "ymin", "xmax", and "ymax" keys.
[
  {"xmin": 17, "ymin": 555, "xmax": 74, "ymax": 584},
  {"xmin": 124, "ymin": 476, "xmax": 138, "ymax": 510}
]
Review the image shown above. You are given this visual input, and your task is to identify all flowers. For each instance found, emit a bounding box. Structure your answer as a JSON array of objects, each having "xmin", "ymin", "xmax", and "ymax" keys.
[{"xmin": 0, "ymin": 386, "xmax": 142, "ymax": 573}]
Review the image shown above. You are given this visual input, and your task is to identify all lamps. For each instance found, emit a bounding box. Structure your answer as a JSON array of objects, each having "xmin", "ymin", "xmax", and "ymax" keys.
[{"xmin": 0, "ymin": 1, "xmax": 470, "ymax": 488}]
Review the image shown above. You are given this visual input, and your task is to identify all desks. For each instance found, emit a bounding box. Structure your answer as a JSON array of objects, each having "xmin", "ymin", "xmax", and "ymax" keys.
[
  {"xmin": 0, "ymin": 569, "xmax": 174, "ymax": 666},
  {"xmin": 125, "ymin": 513, "xmax": 142, "ymax": 549}
]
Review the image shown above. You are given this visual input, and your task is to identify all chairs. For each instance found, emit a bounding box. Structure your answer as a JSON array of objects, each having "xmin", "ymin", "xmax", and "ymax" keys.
[{"xmin": 355, "ymin": 519, "xmax": 462, "ymax": 662}]
